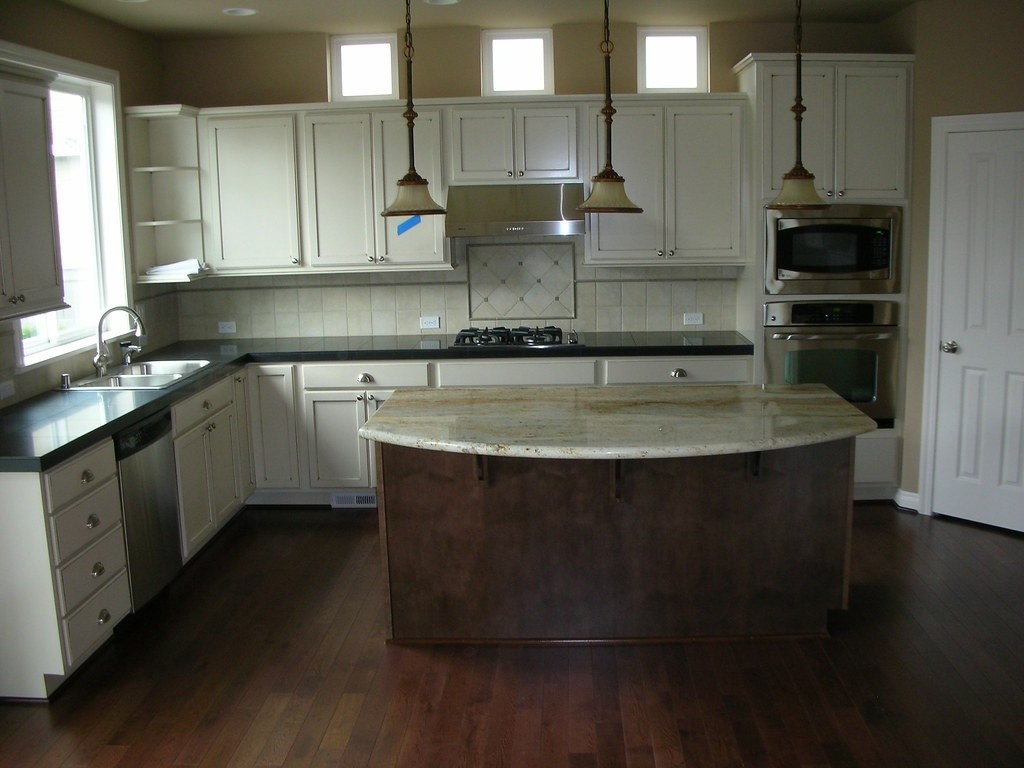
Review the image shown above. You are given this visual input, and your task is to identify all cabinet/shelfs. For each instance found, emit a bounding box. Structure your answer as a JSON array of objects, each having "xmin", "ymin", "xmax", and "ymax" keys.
[
  {"xmin": 606, "ymin": 355, "xmax": 755, "ymax": 385},
  {"xmin": 252, "ymin": 361, "xmax": 301, "ymax": 491},
  {"xmin": 307, "ymin": 361, "xmax": 434, "ymax": 490},
  {"xmin": 172, "ymin": 374, "xmax": 240, "ymax": 564},
  {"xmin": 232, "ymin": 368, "xmax": 256, "ymax": 501},
  {"xmin": 585, "ymin": 91, "xmax": 748, "ymax": 268},
  {"xmin": 122, "ymin": 103, "xmax": 205, "ymax": 283},
  {"xmin": 198, "ymin": 103, "xmax": 307, "ymax": 276},
  {"xmin": 436, "ymin": 359, "xmax": 601, "ymax": 388},
  {"xmin": 731, "ymin": 51, "xmax": 916, "ymax": 205},
  {"xmin": 450, "ymin": 94, "xmax": 584, "ymax": 184},
  {"xmin": 305, "ymin": 97, "xmax": 458, "ymax": 274},
  {"xmin": 0, "ymin": 58, "xmax": 71, "ymax": 323},
  {"xmin": 0, "ymin": 437, "xmax": 133, "ymax": 699}
]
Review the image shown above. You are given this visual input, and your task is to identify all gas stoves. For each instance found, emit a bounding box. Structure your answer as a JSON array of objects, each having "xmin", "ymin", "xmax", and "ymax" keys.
[{"xmin": 454, "ymin": 324, "xmax": 564, "ymax": 348}]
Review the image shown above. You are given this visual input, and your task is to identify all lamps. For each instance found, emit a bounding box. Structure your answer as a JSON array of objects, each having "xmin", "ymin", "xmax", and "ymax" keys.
[
  {"xmin": 575, "ymin": 0, "xmax": 646, "ymax": 214},
  {"xmin": 381, "ymin": 0, "xmax": 450, "ymax": 216},
  {"xmin": 763, "ymin": 0, "xmax": 831, "ymax": 210}
]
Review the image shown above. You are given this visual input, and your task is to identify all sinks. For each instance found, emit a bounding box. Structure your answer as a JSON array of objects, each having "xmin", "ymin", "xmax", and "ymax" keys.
[
  {"xmin": 54, "ymin": 375, "xmax": 190, "ymax": 389},
  {"xmin": 109, "ymin": 359, "xmax": 210, "ymax": 375}
]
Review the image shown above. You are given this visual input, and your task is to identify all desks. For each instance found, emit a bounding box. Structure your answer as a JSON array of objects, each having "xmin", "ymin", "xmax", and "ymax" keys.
[{"xmin": 358, "ymin": 383, "xmax": 879, "ymax": 646}]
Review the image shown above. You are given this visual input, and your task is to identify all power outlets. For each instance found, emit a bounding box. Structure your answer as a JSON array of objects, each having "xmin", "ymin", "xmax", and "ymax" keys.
[
  {"xmin": 683, "ymin": 312, "xmax": 704, "ymax": 325},
  {"xmin": 419, "ymin": 316, "xmax": 441, "ymax": 329},
  {"xmin": 217, "ymin": 321, "xmax": 237, "ymax": 333},
  {"xmin": 0, "ymin": 380, "xmax": 16, "ymax": 401}
]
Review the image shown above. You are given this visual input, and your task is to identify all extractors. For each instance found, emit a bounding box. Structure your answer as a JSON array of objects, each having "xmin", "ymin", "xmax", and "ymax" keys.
[{"xmin": 443, "ymin": 184, "xmax": 586, "ymax": 244}]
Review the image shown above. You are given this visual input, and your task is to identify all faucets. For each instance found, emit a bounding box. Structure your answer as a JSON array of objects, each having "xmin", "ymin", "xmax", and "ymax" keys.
[
  {"xmin": 92, "ymin": 306, "xmax": 146, "ymax": 377},
  {"xmin": 120, "ymin": 340, "xmax": 142, "ymax": 366}
]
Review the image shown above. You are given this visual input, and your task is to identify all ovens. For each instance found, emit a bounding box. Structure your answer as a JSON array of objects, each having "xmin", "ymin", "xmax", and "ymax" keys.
[{"xmin": 762, "ymin": 204, "xmax": 903, "ymax": 431}]
